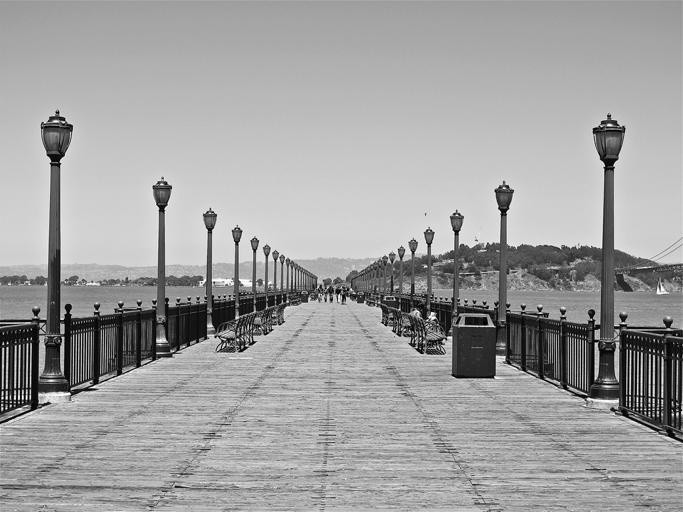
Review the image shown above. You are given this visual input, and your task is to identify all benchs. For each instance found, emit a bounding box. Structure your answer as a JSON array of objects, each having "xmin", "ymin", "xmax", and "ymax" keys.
[
  {"xmin": 213, "ymin": 302, "xmax": 289, "ymax": 354},
  {"xmin": 378, "ymin": 302, "xmax": 447, "ymax": 355}
]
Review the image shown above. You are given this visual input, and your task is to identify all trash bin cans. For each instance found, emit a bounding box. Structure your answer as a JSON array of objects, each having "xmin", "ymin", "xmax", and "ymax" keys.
[
  {"xmin": 357, "ymin": 291, "xmax": 365, "ymax": 303},
  {"xmin": 301, "ymin": 290, "xmax": 309, "ymax": 303},
  {"xmin": 382, "ymin": 295, "xmax": 397, "ymax": 309},
  {"xmin": 451, "ymin": 313, "xmax": 497, "ymax": 378},
  {"xmin": 350, "ymin": 291, "xmax": 357, "ymax": 300}
]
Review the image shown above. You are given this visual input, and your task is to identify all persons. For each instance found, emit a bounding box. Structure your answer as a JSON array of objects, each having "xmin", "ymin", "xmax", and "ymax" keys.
[
  {"xmin": 316, "ymin": 283, "xmax": 351, "ymax": 304},
  {"xmin": 411, "ymin": 302, "xmax": 440, "ymax": 336}
]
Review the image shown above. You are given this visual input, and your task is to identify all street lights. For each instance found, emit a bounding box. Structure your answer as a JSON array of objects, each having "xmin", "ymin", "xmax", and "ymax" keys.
[
  {"xmin": 592, "ymin": 112, "xmax": 626, "ymax": 401},
  {"xmin": 151, "ymin": 175, "xmax": 175, "ymax": 358},
  {"xmin": 37, "ymin": 107, "xmax": 74, "ymax": 394},
  {"xmin": 250, "ymin": 234, "xmax": 318, "ymax": 313},
  {"xmin": 203, "ymin": 206, "xmax": 218, "ymax": 340},
  {"xmin": 493, "ymin": 178, "xmax": 514, "ymax": 358},
  {"xmin": 349, "ymin": 237, "xmax": 418, "ymax": 314},
  {"xmin": 230, "ymin": 224, "xmax": 243, "ymax": 326},
  {"xmin": 423, "ymin": 226, "xmax": 435, "ymax": 318},
  {"xmin": 448, "ymin": 208, "xmax": 465, "ymax": 337}
]
